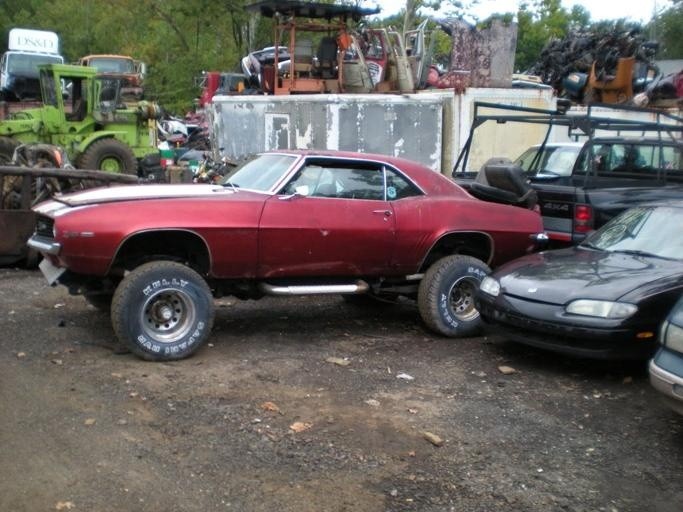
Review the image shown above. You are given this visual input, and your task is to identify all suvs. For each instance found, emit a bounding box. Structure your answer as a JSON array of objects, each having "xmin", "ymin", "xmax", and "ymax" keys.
[{"xmin": 73, "ymin": 54, "xmax": 147, "ymax": 102}]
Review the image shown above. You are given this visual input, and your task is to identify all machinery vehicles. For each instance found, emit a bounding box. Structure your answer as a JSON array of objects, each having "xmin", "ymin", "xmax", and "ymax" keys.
[{"xmin": 0, "ymin": 61, "xmax": 161, "ymax": 268}]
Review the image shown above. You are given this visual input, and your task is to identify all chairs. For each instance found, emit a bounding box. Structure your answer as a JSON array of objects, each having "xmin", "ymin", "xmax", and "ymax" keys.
[
  {"xmin": 315, "ymin": 183, "xmax": 353, "ymax": 198},
  {"xmin": 65, "ymin": 97, "xmax": 87, "ymax": 121},
  {"xmin": 311, "ymin": 35, "xmax": 339, "ymax": 78}
]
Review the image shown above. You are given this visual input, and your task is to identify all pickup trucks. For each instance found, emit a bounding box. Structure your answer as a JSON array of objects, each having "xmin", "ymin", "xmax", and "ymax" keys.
[{"xmin": 510, "ymin": 136, "xmax": 682, "ymax": 247}]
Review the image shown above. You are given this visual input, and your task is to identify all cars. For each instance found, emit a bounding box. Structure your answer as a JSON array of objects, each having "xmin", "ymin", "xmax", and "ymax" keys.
[
  {"xmin": 474, "ymin": 198, "xmax": 683, "ymax": 377},
  {"xmin": 0, "ymin": 51, "xmax": 65, "ymax": 95},
  {"xmin": 26, "ymin": 148, "xmax": 546, "ymax": 363},
  {"xmin": 647, "ymin": 293, "xmax": 683, "ymax": 419},
  {"xmin": 456, "ymin": 140, "xmax": 584, "ymax": 189}
]
